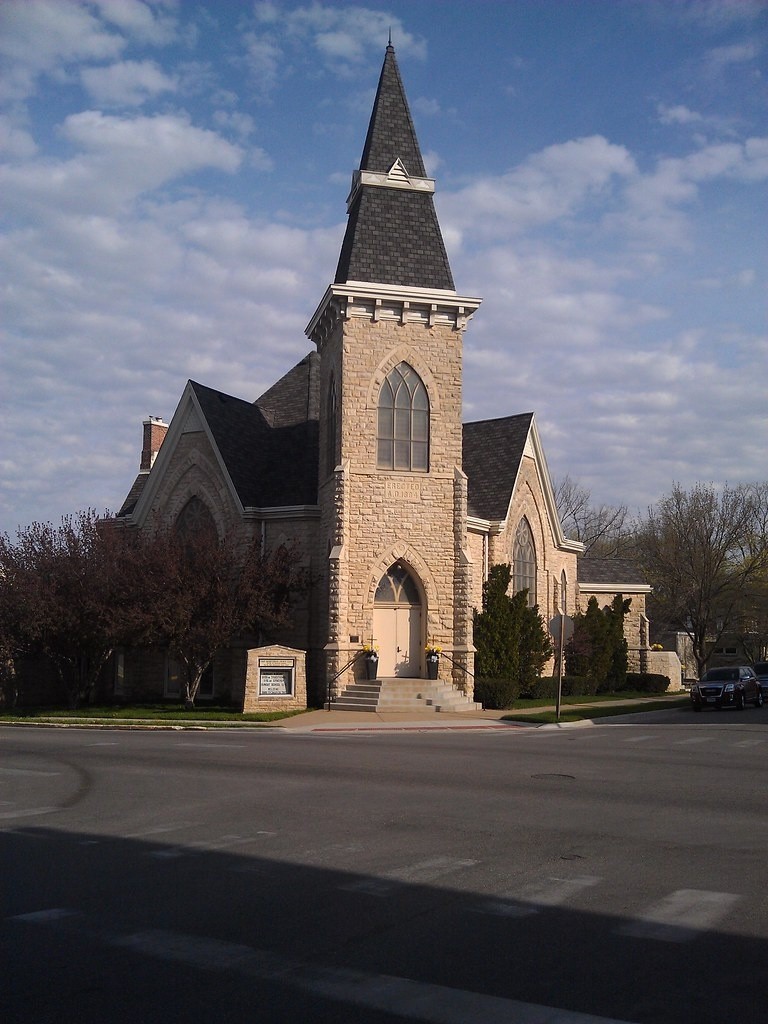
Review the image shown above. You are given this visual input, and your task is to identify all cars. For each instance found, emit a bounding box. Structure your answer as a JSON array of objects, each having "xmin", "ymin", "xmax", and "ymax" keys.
[
  {"xmin": 690, "ymin": 666, "xmax": 764, "ymax": 712},
  {"xmin": 753, "ymin": 662, "xmax": 768, "ymax": 699}
]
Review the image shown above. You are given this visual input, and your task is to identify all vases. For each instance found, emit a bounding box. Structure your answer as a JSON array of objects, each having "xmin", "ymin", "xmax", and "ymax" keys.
[
  {"xmin": 426, "ymin": 652, "xmax": 440, "ymax": 681},
  {"xmin": 365, "ymin": 652, "xmax": 379, "ymax": 680},
  {"xmin": 652, "ymin": 648, "xmax": 661, "ymax": 652}
]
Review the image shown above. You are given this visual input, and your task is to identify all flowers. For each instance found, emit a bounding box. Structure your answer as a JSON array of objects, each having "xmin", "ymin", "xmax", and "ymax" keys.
[
  {"xmin": 424, "ymin": 642, "xmax": 442, "ymax": 654},
  {"xmin": 362, "ymin": 643, "xmax": 380, "ymax": 654},
  {"xmin": 650, "ymin": 643, "xmax": 663, "ymax": 649}
]
